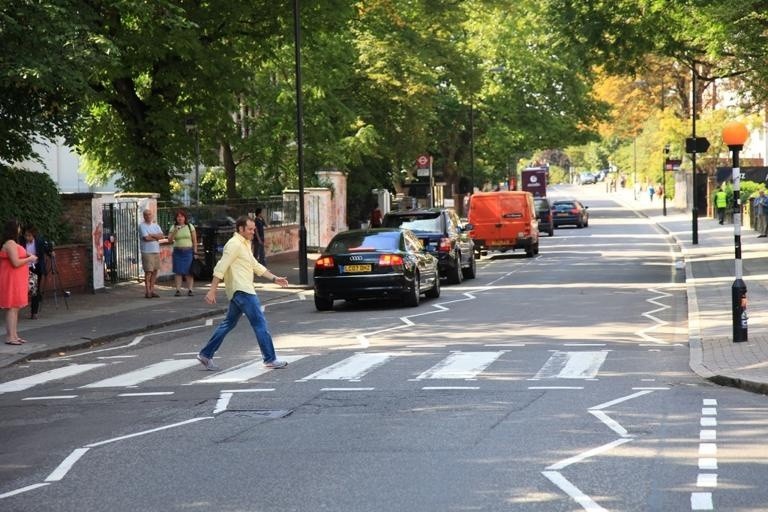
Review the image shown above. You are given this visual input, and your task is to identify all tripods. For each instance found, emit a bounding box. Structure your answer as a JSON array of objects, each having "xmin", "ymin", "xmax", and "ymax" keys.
[{"xmin": 39, "ymin": 254, "xmax": 69, "ymax": 311}]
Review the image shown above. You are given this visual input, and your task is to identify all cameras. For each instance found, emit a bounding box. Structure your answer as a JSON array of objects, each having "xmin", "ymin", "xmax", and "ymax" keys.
[{"xmin": 42, "ymin": 239, "xmax": 55, "ymax": 252}]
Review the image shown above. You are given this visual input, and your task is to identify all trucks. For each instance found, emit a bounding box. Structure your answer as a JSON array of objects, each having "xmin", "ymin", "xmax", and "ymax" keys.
[{"xmin": 466, "ymin": 191, "xmax": 540, "ymax": 259}]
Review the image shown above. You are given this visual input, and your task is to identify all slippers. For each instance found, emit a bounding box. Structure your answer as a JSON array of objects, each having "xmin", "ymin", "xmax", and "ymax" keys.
[
  {"xmin": 5, "ymin": 340, "xmax": 22, "ymax": 345},
  {"xmin": 17, "ymin": 338, "xmax": 27, "ymax": 344}
]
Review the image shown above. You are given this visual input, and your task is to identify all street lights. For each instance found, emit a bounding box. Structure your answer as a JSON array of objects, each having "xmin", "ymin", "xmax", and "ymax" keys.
[{"xmin": 720, "ymin": 122, "xmax": 751, "ymax": 343}]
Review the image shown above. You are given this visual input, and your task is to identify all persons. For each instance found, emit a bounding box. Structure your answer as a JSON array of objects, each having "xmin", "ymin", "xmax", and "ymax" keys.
[
  {"xmin": 0, "ymin": 222, "xmax": 38, "ymax": 344},
  {"xmin": 754, "ymin": 192, "xmax": 768, "ymax": 236},
  {"xmin": 714, "ymin": 187, "xmax": 729, "ymax": 223},
  {"xmin": 137, "ymin": 209, "xmax": 164, "ymax": 296},
  {"xmin": 371, "ymin": 203, "xmax": 383, "ymax": 227},
  {"xmin": 20, "ymin": 226, "xmax": 57, "ymax": 321},
  {"xmin": 609, "ymin": 173, "xmax": 661, "ymax": 200},
  {"xmin": 255, "ymin": 208, "xmax": 268, "ymax": 261},
  {"xmin": 196, "ymin": 214, "xmax": 288, "ymax": 369},
  {"xmin": 167, "ymin": 214, "xmax": 198, "ymax": 295}
]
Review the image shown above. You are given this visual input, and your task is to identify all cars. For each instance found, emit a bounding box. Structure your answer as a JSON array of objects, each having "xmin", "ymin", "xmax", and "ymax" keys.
[
  {"xmin": 576, "ymin": 172, "xmax": 596, "ymax": 185},
  {"xmin": 549, "ymin": 200, "xmax": 589, "ymax": 229},
  {"xmin": 312, "ymin": 227, "xmax": 441, "ymax": 311},
  {"xmin": 533, "ymin": 198, "xmax": 553, "ymax": 236}
]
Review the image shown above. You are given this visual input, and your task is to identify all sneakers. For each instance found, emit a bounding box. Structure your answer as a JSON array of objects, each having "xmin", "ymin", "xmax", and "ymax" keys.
[
  {"xmin": 187, "ymin": 290, "xmax": 194, "ymax": 296},
  {"xmin": 263, "ymin": 359, "xmax": 288, "ymax": 369},
  {"xmin": 196, "ymin": 354, "xmax": 220, "ymax": 371},
  {"xmin": 174, "ymin": 290, "xmax": 182, "ymax": 297},
  {"xmin": 145, "ymin": 293, "xmax": 153, "ymax": 298},
  {"xmin": 153, "ymin": 292, "xmax": 161, "ymax": 298}
]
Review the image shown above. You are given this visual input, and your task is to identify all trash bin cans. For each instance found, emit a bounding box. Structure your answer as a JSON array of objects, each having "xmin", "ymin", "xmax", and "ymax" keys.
[{"xmin": 202, "ymin": 229, "xmax": 236, "ymax": 280}]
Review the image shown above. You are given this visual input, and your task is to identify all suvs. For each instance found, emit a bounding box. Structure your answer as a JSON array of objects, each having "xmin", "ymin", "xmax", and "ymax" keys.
[{"xmin": 381, "ymin": 209, "xmax": 478, "ymax": 283}]
